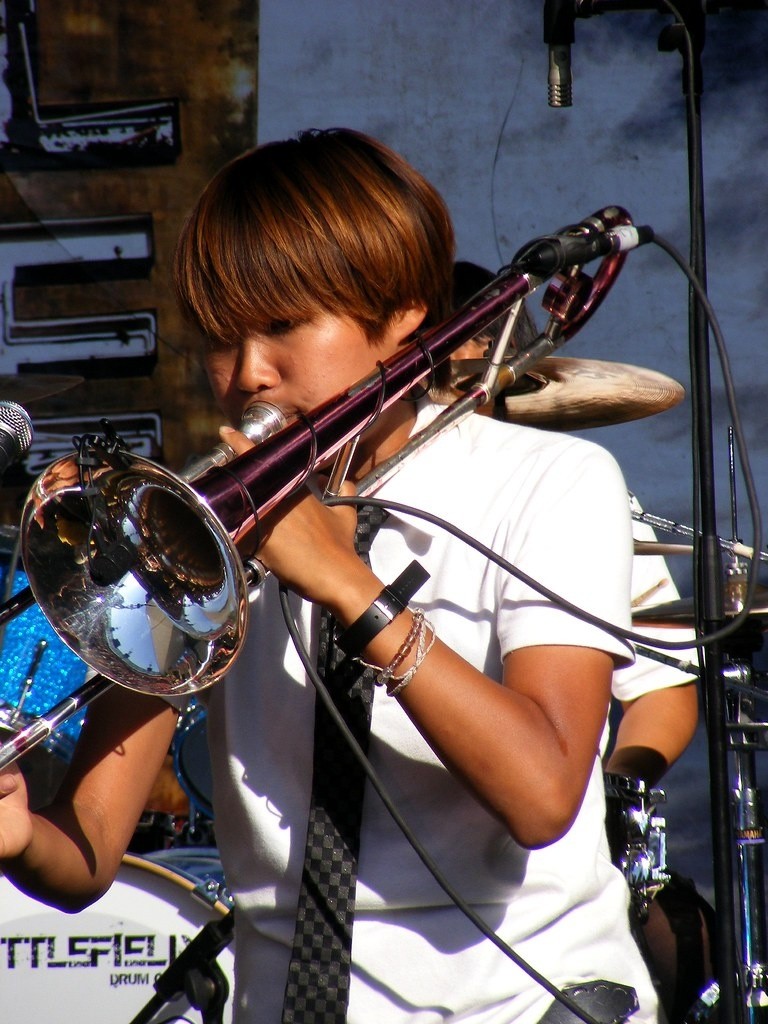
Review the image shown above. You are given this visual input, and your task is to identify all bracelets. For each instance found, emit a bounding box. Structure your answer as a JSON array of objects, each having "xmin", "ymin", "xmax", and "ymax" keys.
[
  {"xmin": 360, "ymin": 620, "xmax": 436, "ymax": 697},
  {"xmin": 333, "ymin": 561, "xmax": 430, "ymax": 656},
  {"xmin": 375, "ymin": 608, "xmax": 425, "ymax": 685}
]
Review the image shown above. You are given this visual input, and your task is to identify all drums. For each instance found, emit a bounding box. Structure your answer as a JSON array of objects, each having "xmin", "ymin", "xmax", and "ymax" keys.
[
  {"xmin": 604, "ymin": 774, "xmax": 652, "ymax": 884},
  {"xmin": 2, "ymin": 848, "xmax": 239, "ymax": 1024}
]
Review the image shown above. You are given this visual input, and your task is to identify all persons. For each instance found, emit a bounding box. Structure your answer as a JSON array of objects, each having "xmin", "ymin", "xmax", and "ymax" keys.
[{"xmin": 1, "ymin": 130, "xmax": 721, "ymax": 1024}]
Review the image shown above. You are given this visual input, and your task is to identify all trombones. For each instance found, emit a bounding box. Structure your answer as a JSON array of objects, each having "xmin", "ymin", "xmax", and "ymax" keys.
[{"xmin": 0, "ymin": 199, "xmax": 643, "ymax": 773}]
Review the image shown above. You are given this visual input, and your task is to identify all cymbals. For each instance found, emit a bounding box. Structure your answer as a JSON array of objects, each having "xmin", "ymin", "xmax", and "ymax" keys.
[
  {"xmin": 631, "ymin": 580, "xmax": 768, "ymax": 626},
  {"xmin": 417, "ymin": 352, "xmax": 687, "ymax": 432}
]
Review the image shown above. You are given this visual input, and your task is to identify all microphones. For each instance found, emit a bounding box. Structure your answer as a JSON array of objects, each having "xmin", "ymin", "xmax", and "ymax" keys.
[
  {"xmin": 89, "ymin": 538, "xmax": 138, "ymax": 586},
  {"xmin": 534, "ymin": 225, "xmax": 655, "ymax": 272},
  {"xmin": 543, "ymin": 0, "xmax": 573, "ymax": 107},
  {"xmin": 0, "ymin": 399, "xmax": 34, "ymax": 476}
]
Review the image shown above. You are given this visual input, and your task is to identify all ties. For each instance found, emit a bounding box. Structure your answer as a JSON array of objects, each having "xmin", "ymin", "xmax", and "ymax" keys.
[{"xmin": 280, "ymin": 498, "xmax": 391, "ymax": 1024}]
